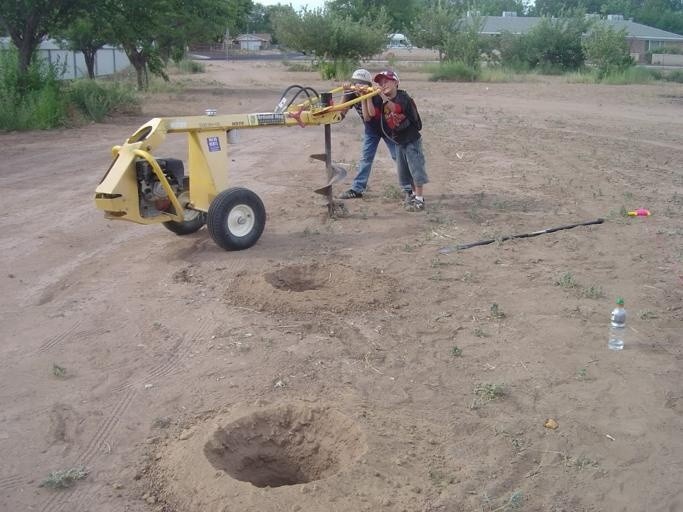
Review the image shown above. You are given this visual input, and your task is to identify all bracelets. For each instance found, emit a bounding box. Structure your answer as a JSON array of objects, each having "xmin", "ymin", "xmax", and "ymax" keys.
[{"xmin": 383, "ymin": 97, "xmax": 390, "ymax": 104}]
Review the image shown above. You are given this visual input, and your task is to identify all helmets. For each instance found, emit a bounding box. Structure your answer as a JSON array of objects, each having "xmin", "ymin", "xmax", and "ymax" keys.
[{"xmin": 351, "ymin": 68, "xmax": 373, "ymax": 84}]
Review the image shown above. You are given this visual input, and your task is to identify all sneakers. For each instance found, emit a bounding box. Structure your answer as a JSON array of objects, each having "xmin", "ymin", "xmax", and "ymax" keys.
[
  {"xmin": 338, "ymin": 188, "xmax": 363, "ymax": 199},
  {"xmin": 404, "ymin": 194, "xmax": 425, "ymax": 213}
]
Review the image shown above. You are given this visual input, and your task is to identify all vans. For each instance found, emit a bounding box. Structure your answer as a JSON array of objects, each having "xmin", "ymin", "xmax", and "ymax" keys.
[{"xmin": 384, "ymin": 33, "xmax": 413, "ymax": 48}]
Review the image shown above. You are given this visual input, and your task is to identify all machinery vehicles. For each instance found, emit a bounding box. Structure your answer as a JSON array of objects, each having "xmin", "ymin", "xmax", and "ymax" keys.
[{"xmin": 99, "ymin": 82, "xmax": 383, "ymax": 253}]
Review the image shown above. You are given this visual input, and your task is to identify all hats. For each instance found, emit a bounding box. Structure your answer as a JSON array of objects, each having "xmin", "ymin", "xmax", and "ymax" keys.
[{"xmin": 374, "ymin": 70, "xmax": 400, "ymax": 85}]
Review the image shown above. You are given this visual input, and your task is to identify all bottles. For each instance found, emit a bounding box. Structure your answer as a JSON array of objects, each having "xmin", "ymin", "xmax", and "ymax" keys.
[{"xmin": 608, "ymin": 300, "xmax": 629, "ymax": 353}]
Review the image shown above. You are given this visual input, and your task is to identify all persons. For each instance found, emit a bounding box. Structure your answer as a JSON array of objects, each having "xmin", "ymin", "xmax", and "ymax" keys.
[
  {"xmin": 338, "ymin": 69, "xmax": 413, "ymax": 199},
  {"xmin": 365, "ymin": 70, "xmax": 429, "ymax": 212}
]
